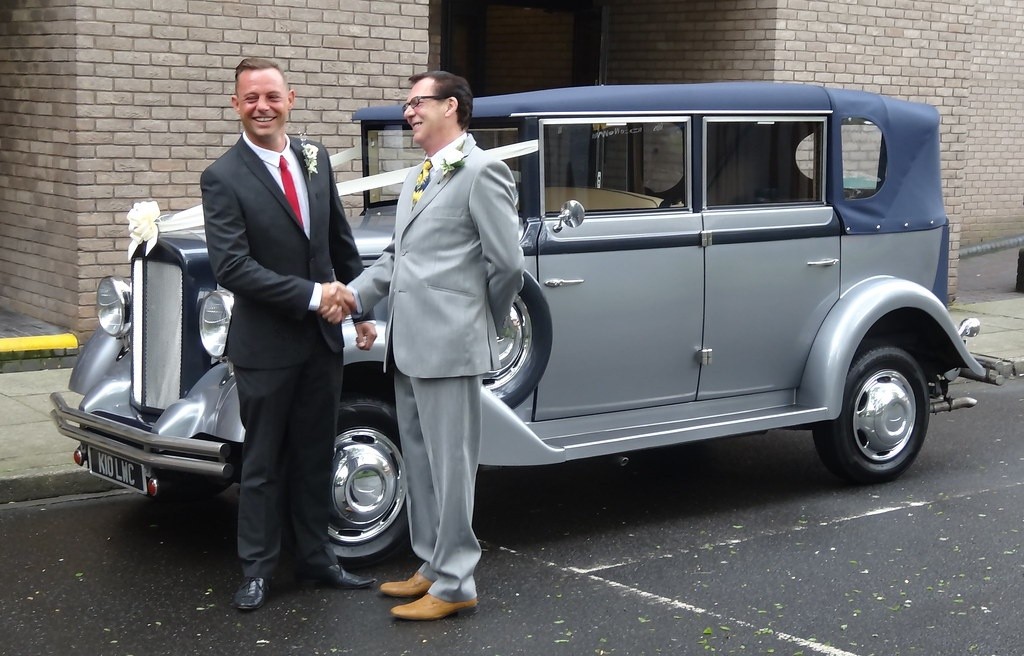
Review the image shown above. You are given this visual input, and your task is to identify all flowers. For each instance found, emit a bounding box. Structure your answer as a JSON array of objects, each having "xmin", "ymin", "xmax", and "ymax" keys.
[
  {"xmin": 126, "ymin": 200, "xmax": 161, "ymax": 262},
  {"xmin": 298, "ymin": 131, "xmax": 319, "ymax": 181},
  {"xmin": 436, "ymin": 139, "xmax": 466, "ymax": 185}
]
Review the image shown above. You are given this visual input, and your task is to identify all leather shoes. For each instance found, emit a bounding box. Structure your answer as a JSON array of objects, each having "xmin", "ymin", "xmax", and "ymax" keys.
[
  {"xmin": 380, "ymin": 571, "xmax": 432, "ymax": 597},
  {"xmin": 390, "ymin": 591, "xmax": 478, "ymax": 620},
  {"xmin": 232, "ymin": 574, "xmax": 272, "ymax": 608},
  {"xmin": 295, "ymin": 561, "xmax": 378, "ymax": 589}
]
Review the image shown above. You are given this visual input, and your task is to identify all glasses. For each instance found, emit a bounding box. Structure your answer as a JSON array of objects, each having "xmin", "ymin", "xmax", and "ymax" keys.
[{"xmin": 402, "ymin": 95, "xmax": 448, "ymax": 115}]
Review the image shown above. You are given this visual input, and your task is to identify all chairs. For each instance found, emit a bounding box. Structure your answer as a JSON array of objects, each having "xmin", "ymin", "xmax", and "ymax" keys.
[{"xmin": 545, "ymin": 186, "xmax": 662, "ymax": 212}]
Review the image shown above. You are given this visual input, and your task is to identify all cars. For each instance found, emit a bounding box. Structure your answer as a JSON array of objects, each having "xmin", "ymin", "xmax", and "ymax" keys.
[{"xmin": 50, "ymin": 80, "xmax": 1003, "ymax": 575}]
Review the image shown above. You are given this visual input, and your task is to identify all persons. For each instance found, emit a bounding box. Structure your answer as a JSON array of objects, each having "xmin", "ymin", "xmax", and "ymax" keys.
[
  {"xmin": 318, "ymin": 71, "xmax": 525, "ymax": 621},
  {"xmin": 201, "ymin": 58, "xmax": 377, "ymax": 610}
]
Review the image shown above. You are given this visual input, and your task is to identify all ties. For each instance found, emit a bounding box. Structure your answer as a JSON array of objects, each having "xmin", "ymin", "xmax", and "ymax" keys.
[
  {"xmin": 279, "ymin": 156, "xmax": 304, "ymax": 229},
  {"xmin": 411, "ymin": 159, "xmax": 433, "ymax": 211}
]
